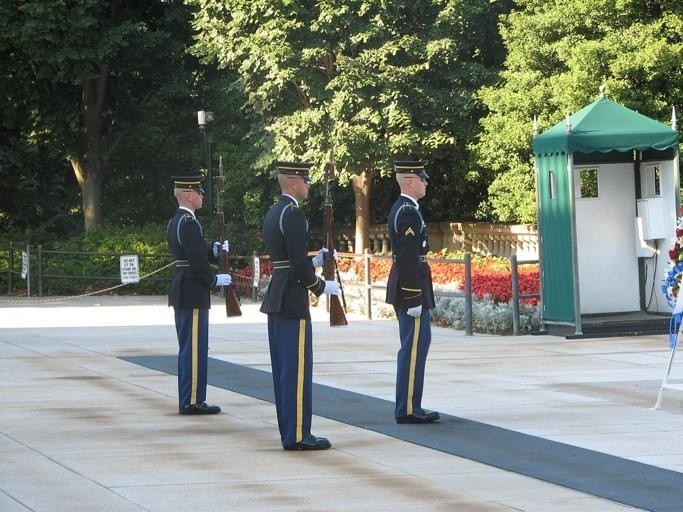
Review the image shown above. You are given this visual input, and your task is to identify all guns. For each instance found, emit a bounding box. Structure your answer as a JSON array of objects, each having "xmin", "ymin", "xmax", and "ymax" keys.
[
  {"xmin": 212, "ymin": 154, "xmax": 243, "ymax": 317},
  {"xmin": 319, "ymin": 137, "xmax": 348, "ymax": 328}
]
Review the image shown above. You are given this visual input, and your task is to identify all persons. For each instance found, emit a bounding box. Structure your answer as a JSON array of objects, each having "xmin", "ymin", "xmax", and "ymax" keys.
[
  {"xmin": 166, "ymin": 175, "xmax": 233, "ymax": 415},
  {"xmin": 384, "ymin": 159, "xmax": 441, "ymax": 424},
  {"xmin": 259, "ymin": 162, "xmax": 342, "ymax": 451}
]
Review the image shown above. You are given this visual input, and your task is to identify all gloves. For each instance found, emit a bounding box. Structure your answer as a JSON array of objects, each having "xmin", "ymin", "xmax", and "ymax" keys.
[
  {"xmin": 323, "ymin": 280, "xmax": 342, "ymax": 296},
  {"xmin": 312, "ymin": 248, "xmax": 339, "ymax": 269},
  {"xmin": 406, "ymin": 305, "xmax": 422, "ymax": 318},
  {"xmin": 213, "ymin": 239, "xmax": 229, "ymax": 258},
  {"xmin": 215, "ymin": 274, "xmax": 232, "ymax": 287}
]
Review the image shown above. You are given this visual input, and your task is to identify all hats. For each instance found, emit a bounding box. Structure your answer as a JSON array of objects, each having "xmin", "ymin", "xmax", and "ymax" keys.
[
  {"xmin": 393, "ymin": 160, "xmax": 431, "ymax": 180},
  {"xmin": 170, "ymin": 176, "xmax": 206, "ymax": 194},
  {"xmin": 277, "ymin": 161, "xmax": 315, "ymax": 182}
]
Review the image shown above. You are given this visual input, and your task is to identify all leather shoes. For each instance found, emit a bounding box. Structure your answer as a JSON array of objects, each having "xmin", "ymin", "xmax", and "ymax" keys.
[
  {"xmin": 395, "ymin": 411, "xmax": 439, "ymax": 424},
  {"xmin": 284, "ymin": 434, "xmax": 330, "ymax": 450},
  {"xmin": 179, "ymin": 401, "xmax": 221, "ymax": 415}
]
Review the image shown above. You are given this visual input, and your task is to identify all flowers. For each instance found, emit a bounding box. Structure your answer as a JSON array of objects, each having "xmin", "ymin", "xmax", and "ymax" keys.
[{"xmin": 663, "ymin": 202, "xmax": 683, "ymax": 307}]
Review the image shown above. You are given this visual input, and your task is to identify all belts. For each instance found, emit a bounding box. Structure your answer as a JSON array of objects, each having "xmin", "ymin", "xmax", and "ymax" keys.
[
  {"xmin": 392, "ymin": 254, "xmax": 427, "ymax": 263},
  {"xmin": 174, "ymin": 260, "xmax": 191, "ymax": 267},
  {"xmin": 271, "ymin": 261, "xmax": 292, "ymax": 270}
]
